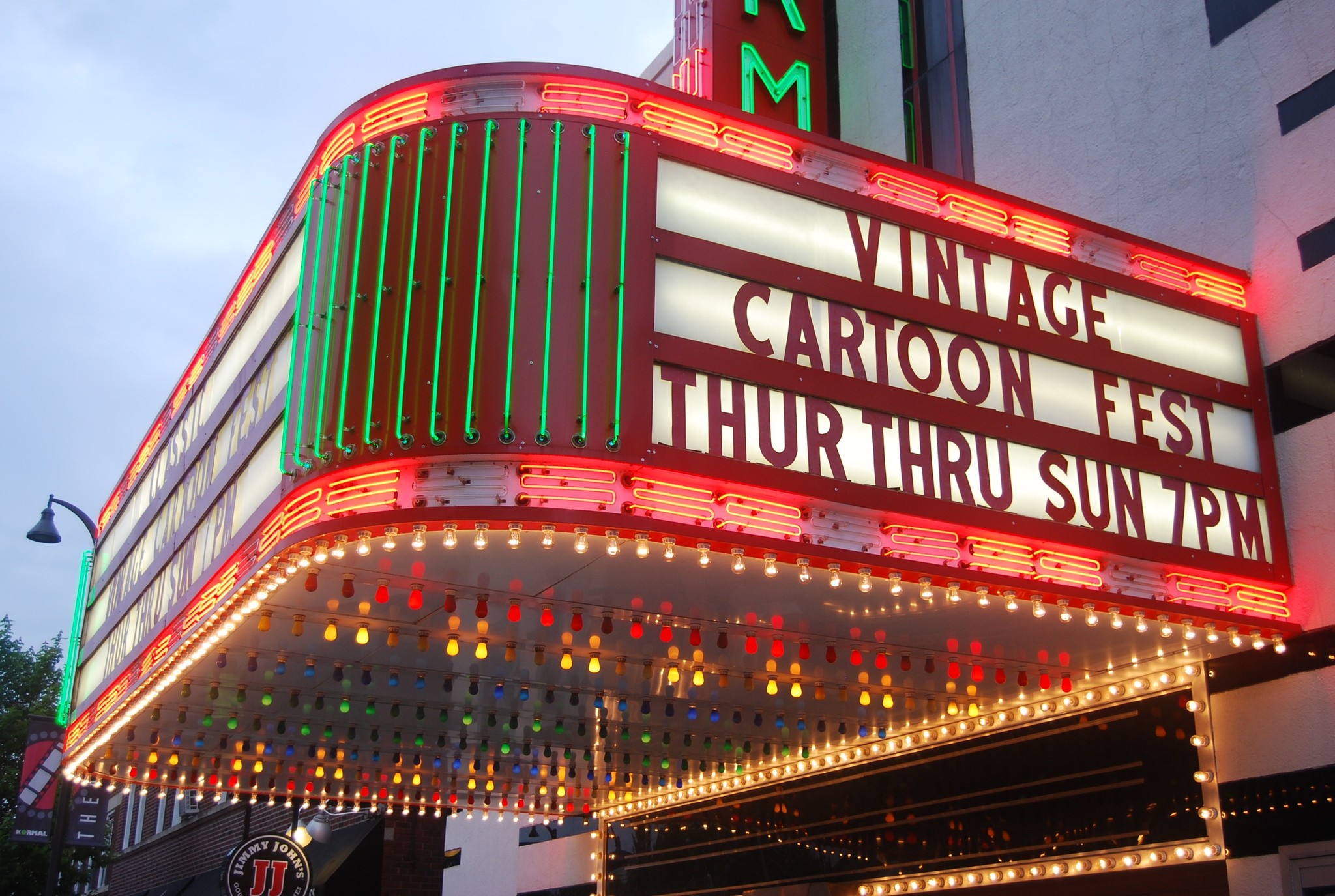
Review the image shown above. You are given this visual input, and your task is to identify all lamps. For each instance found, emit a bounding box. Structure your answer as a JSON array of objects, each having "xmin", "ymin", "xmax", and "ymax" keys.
[{"xmin": 286, "ymin": 804, "xmax": 385, "ymax": 849}]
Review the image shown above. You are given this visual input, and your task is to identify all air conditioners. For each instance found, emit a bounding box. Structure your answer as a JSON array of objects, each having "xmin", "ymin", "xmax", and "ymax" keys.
[{"xmin": 179, "ymin": 789, "xmax": 198, "ymax": 816}]
